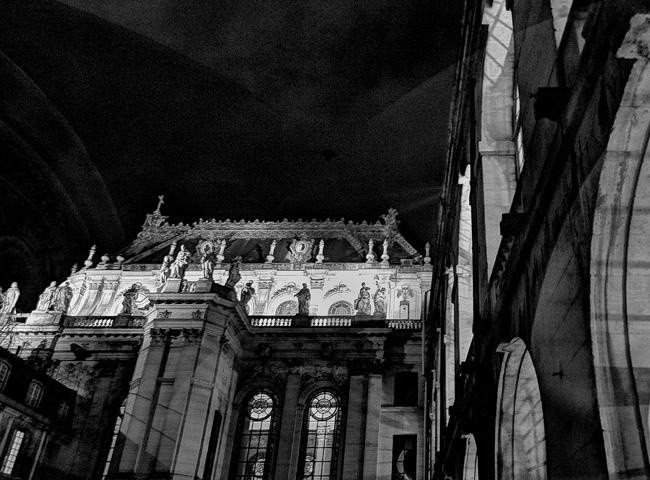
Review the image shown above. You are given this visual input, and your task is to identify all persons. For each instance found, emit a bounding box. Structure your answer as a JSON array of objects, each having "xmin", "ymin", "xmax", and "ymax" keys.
[{"xmin": 1, "ymin": 240, "xmax": 388, "ymax": 321}]
